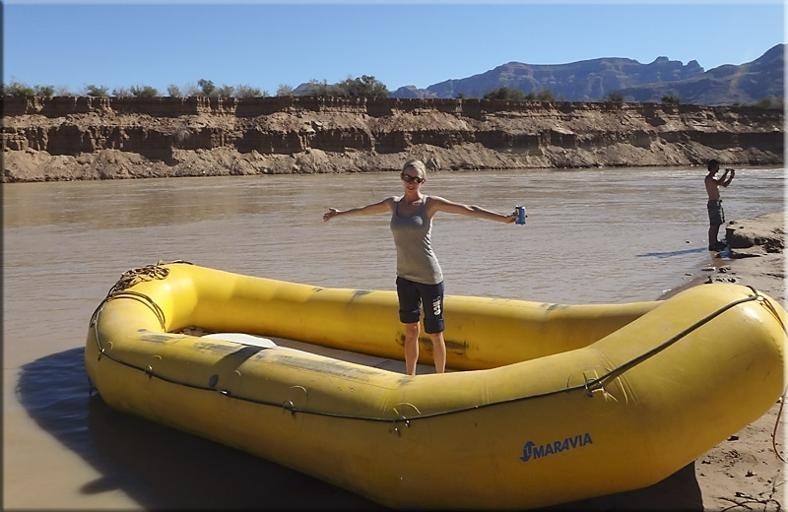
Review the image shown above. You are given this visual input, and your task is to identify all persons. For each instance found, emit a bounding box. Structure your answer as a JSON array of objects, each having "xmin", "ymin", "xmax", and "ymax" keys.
[
  {"xmin": 705, "ymin": 159, "xmax": 736, "ymax": 251},
  {"xmin": 323, "ymin": 158, "xmax": 528, "ymax": 374}
]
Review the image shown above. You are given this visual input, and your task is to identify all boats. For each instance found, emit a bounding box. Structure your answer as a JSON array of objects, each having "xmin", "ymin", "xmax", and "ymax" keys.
[{"xmin": 84, "ymin": 260, "xmax": 787, "ymax": 510}]
